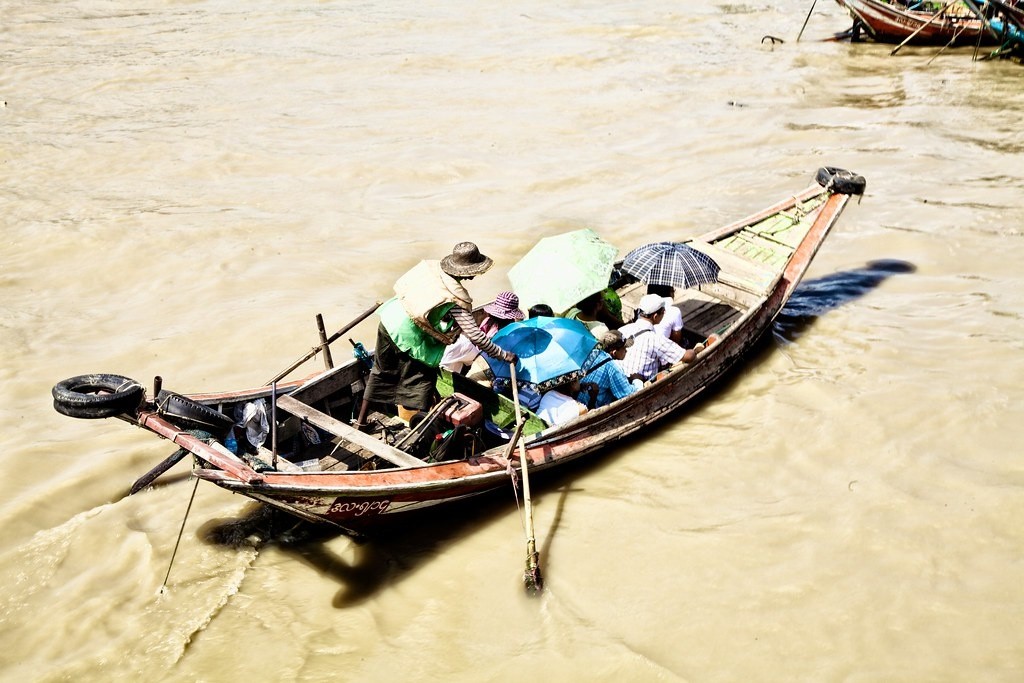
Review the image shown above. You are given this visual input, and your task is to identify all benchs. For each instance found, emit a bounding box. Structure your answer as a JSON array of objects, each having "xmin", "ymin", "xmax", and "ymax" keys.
[{"xmin": 432, "ymin": 365, "xmax": 546, "ymax": 437}]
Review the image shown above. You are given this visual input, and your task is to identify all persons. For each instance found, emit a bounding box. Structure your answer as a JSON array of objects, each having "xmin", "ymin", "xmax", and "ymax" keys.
[
  {"xmin": 535, "ymin": 375, "xmax": 599, "ymax": 429},
  {"xmin": 439, "ymin": 324, "xmax": 480, "ymax": 380},
  {"xmin": 610, "ymin": 294, "xmax": 705, "ymax": 382},
  {"xmin": 576, "ymin": 330, "xmax": 644, "ymax": 413},
  {"xmin": 527, "ymin": 304, "xmax": 553, "ymax": 319},
  {"xmin": 480, "ymin": 292, "xmax": 525, "ymax": 342},
  {"xmin": 482, "ymin": 380, "xmax": 541, "ymax": 436},
  {"xmin": 568, "ymin": 290, "xmax": 612, "ymax": 341},
  {"xmin": 356, "ymin": 242, "xmax": 518, "ymax": 423},
  {"xmin": 647, "ymin": 285, "xmax": 684, "ymax": 341}
]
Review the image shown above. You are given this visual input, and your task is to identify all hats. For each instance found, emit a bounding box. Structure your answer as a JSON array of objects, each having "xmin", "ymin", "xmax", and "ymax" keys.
[
  {"xmin": 441, "ymin": 241, "xmax": 495, "ymax": 277},
  {"xmin": 483, "ymin": 292, "xmax": 525, "ymax": 320},
  {"xmin": 601, "ymin": 330, "xmax": 634, "ymax": 349},
  {"xmin": 638, "ymin": 293, "xmax": 673, "ymax": 316}
]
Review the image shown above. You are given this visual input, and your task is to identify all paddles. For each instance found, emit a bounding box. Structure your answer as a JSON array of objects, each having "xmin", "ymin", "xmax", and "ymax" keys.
[
  {"xmin": 127, "ymin": 298, "xmax": 383, "ymax": 496},
  {"xmin": 891, "ymin": 0, "xmax": 957, "ymax": 57},
  {"xmin": 509, "ymin": 361, "xmax": 544, "ymax": 600}
]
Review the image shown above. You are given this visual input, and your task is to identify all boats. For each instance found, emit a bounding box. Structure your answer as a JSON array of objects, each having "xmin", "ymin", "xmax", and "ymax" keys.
[
  {"xmin": 52, "ymin": 168, "xmax": 865, "ymax": 539},
  {"xmin": 839, "ymin": 0, "xmax": 1024, "ymax": 67}
]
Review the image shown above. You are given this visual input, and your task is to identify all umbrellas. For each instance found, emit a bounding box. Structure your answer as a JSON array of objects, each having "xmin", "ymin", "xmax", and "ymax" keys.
[
  {"xmin": 506, "ymin": 228, "xmax": 619, "ymax": 313},
  {"xmin": 481, "ymin": 316, "xmax": 603, "ymax": 393},
  {"xmin": 622, "ymin": 242, "xmax": 721, "ymax": 291}
]
